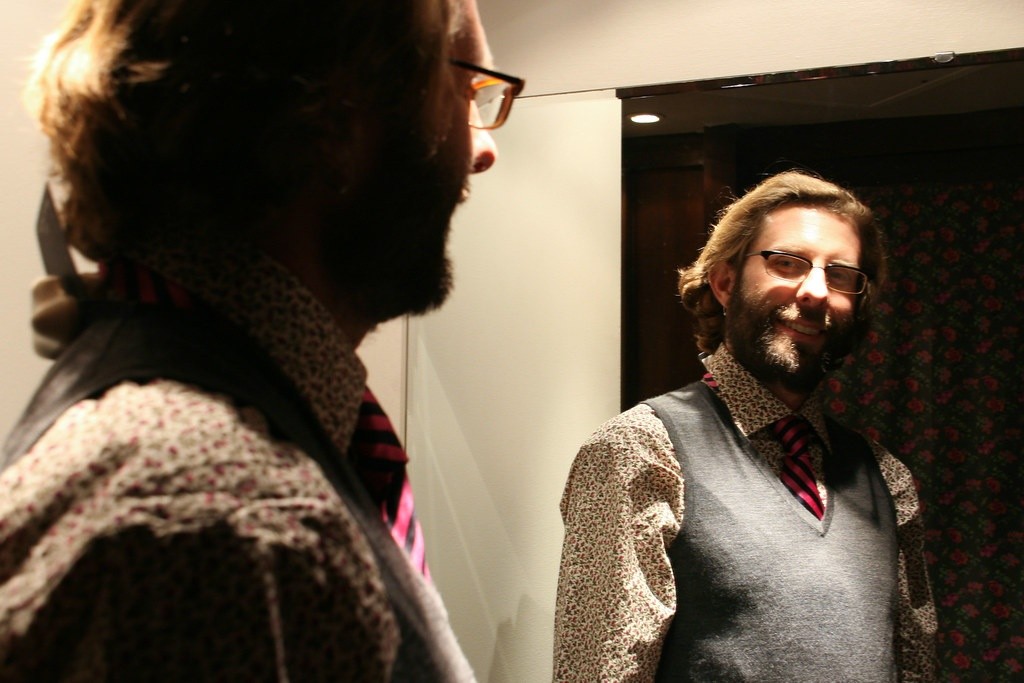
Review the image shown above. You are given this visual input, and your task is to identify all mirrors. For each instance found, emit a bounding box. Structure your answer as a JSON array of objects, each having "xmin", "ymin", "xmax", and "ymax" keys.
[{"xmin": 402, "ymin": 47, "xmax": 1024, "ymax": 683}]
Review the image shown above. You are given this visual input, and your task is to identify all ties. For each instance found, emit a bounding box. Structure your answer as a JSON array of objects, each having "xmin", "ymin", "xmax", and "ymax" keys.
[
  {"xmin": 704, "ymin": 372, "xmax": 825, "ymax": 521},
  {"xmin": 351, "ymin": 383, "xmax": 431, "ymax": 586}
]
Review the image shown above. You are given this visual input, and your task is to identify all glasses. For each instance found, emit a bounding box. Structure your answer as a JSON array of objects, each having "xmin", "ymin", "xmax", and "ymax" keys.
[
  {"xmin": 745, "ymin": 250, "xmax": 872, "ymax": 296},
  {"xmin": 451, "ymin": 55, "xmax": 526, "ymax": 132}
]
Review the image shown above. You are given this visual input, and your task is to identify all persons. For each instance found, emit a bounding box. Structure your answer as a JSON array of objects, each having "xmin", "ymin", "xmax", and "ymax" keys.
[
  {"xmin": 1, "ymin": 1, "xmax": 499, "ymax": 682},
  {"xmin": 552, "ymin": 171, "xmax": 937, "ymax": 683}
]
